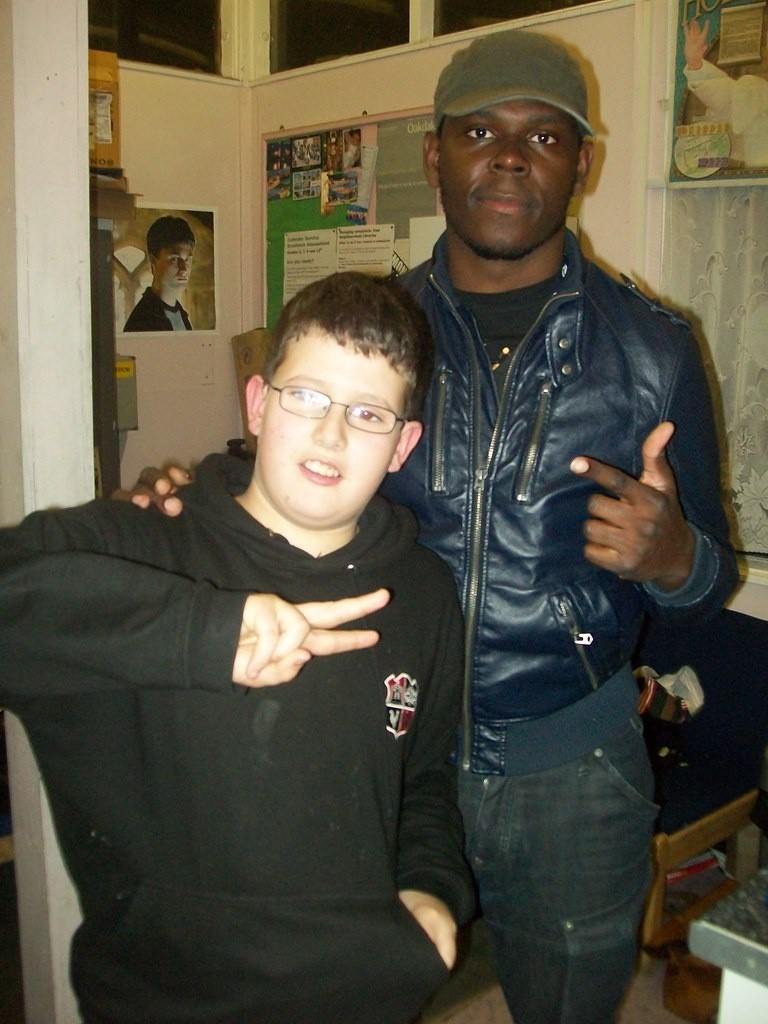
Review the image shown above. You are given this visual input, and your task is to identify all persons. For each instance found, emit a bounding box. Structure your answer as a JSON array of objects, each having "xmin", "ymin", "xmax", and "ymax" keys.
[
  {"xmin": 0, "ymin": 271, "xmax": 514, "ymax": 1024},
  {"xmin": 269, "ymin": 128, "xmax": 360, "ymax": 196},
  {"xmin": 681, "ymin": 19, "xmax": 768, "ymax": 170},
  {"xmin": 107, "ymin": 28, "xmax": 741, "ymax": 1024},
  {"xmin": 123, "ymin": 216, "xmax": 196, "ymax": 330}
]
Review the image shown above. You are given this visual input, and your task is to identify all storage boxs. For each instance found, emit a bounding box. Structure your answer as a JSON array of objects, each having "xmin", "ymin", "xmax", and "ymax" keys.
[
  {"xmin": 89, "ymin": 48, "xmax": 121, "ymax": 167},
  {"xmin": 93, "ymin": 352, "xmax": 138, "ymax": 430}
]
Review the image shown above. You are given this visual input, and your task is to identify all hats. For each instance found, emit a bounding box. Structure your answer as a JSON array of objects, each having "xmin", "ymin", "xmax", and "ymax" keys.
[{"xmin": 434, "ymin": 31, "xmax": 597, "ymax": 137}]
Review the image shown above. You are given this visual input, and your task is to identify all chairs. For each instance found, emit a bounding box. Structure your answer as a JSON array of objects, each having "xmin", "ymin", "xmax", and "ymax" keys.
[{"xmin": 626, "ymin": 609, "xmax": 768, "ymax": 974}]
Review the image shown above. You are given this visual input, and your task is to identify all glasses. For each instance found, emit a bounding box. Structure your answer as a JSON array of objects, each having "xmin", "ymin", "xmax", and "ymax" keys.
[{"xmin": 264, "ymin": 382, "xmax": 408, "ymax": 434}]
